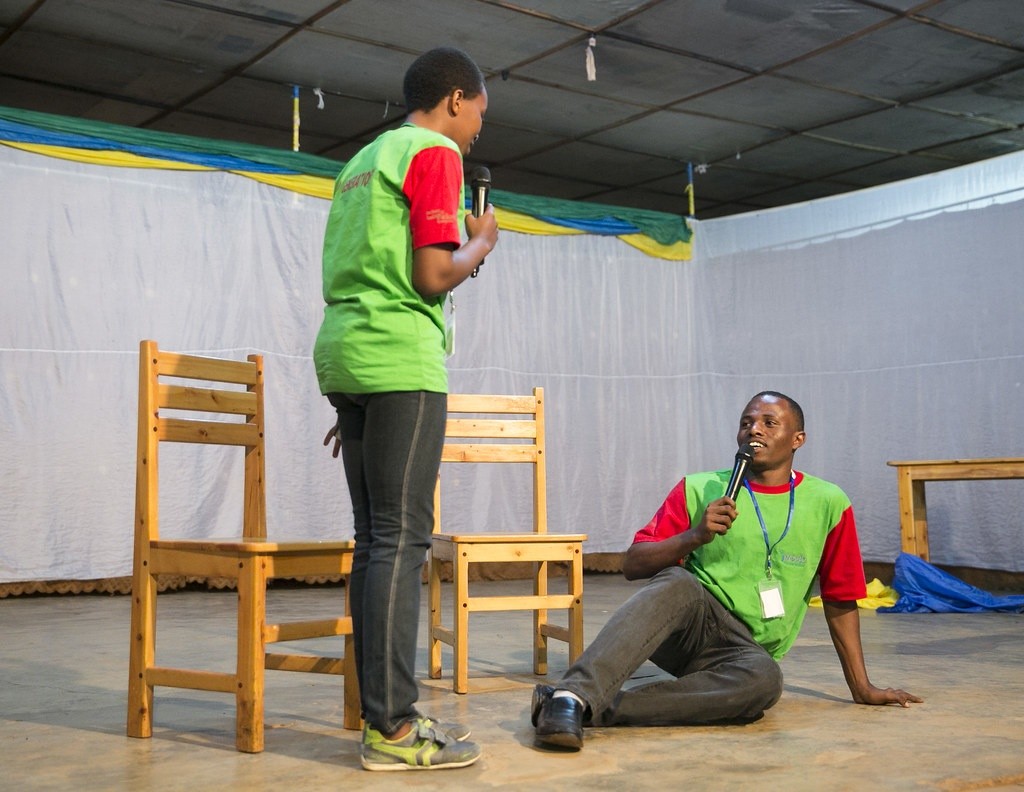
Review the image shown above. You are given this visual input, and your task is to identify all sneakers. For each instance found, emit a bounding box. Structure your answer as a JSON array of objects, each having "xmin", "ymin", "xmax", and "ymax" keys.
[
  {"xmin": 355, "ymin": 706, "xmax": 471, "ymax": 749},
  {"xmin": 358, "ymin": 718, "xmax": 481, "ymax": 775}
]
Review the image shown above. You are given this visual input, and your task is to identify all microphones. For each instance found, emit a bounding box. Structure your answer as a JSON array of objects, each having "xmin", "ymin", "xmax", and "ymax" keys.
[
  {"xmin": 723, "ymin": 443, "xmax": 754, "ymax": 501},
  {"xmin": 470, "ymin": 165, "xmax": 490, "ymax": 278}
]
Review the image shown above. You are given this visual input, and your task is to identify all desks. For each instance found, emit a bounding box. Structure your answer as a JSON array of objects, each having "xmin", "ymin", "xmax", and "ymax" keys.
[{"xmin": 886, "ymin": 458, "xmax": 1024, "ymax": 557}]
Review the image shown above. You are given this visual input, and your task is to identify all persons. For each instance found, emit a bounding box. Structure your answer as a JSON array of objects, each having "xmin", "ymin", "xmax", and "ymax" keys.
[
  {"xmin": 528, "ymin": 386, "xmax": 926, "ymax": 751},
  {"xmin": 308, "ymin": 45, "xmax": 489, "ymax": 775}
]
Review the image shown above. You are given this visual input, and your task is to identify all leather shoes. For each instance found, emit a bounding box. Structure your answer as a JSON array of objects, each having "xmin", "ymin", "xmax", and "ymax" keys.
[
  {"xmin": 537, "ymin": 694, "xmax": 588, "ymax": 755},
  {"xmin": 529, "ymin": 681, "xmax": 566, "ymax": 724}
]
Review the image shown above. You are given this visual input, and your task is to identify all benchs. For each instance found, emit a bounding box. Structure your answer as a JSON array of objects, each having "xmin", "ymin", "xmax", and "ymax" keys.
[
  {"xmin": 125, "ymin": 340, "xmax": 367, "ymax": 756},
  {"xmin": 425, "ymin": 385, "xmax": 588, "ymax": 696}
]
